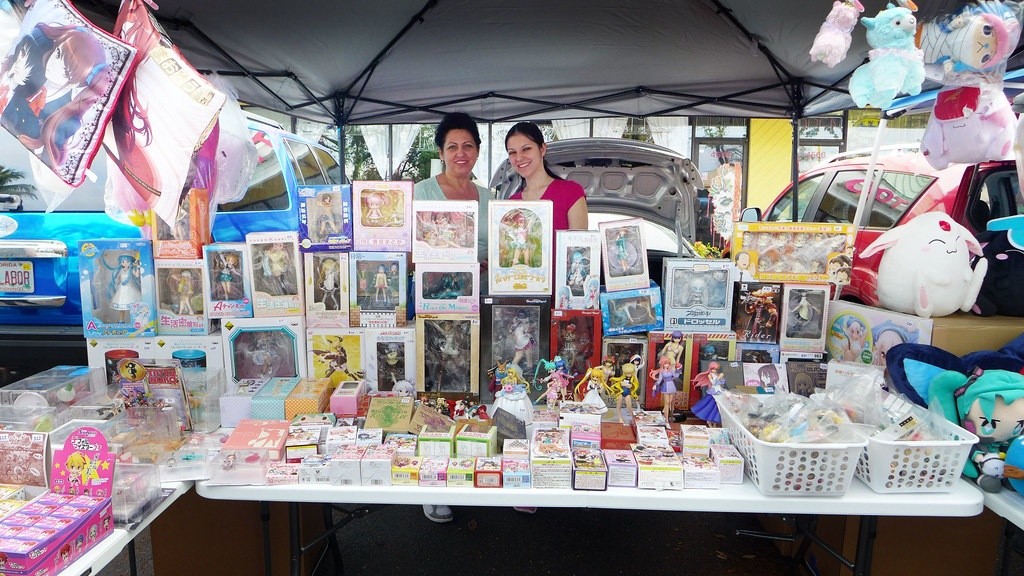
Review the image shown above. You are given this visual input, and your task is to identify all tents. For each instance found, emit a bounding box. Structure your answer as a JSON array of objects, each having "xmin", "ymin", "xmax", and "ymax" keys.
[{"xmin": 1, "ymin": 0, "xmax": 1024, "ymax": 224}]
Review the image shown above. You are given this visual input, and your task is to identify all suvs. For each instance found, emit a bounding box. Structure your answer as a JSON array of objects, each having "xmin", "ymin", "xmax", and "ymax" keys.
[{"xmin": 0, "ymin": 109, "xmax": 353, "ymax": 348}]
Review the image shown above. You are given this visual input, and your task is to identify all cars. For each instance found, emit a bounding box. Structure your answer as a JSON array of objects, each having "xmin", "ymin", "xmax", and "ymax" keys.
[
  {"xmin": 722, "ymin": 143, "xmax": 1023, "ymax": 319},
  {"xmin": 489, "ymin": 136, "xmax": 708, "ymax": 294}
]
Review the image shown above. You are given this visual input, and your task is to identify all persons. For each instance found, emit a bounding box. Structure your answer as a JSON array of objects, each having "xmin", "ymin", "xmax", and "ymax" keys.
[
  {"xmin": 489, "ymin": 355, "xmax": 645, "ymax": 424},
  {"xmin": 650, "ymin": 329, "xmax": 684, "ymax": 429},
  {"xmin": 262, "ymin": 191, "xmax": 528, "ymax": 310},
  {"xmin": 564, "ymin": 323, "xmax": 577, "ymax": 349},
  {"xmin": 508, "ymin": 309, "xmax": 534, "ymax": 367},
  {"xmin": 171, "ymin": 270, "xmax": 195, "ymax": 315},
  {"xmin": 218, "ymin": 253, "xmax": 243, "ymax": 300},
  {"xmin": 690, "ymin": 361, "xmax": 725, "ymax": 427},
  {"xmin": 102, "ymin": 254, "xmax": 141, "ymax": 324},
  {"xmin": 224, "ymin": 454, "xmax": 235, "ymax": 471},
  {"xmin": 616, "ymin": 228, "xmax": 631, "ymax": 275},
  {"xmin": 414, "ymin": 111, "xmax": 496, "ymax": 522},
  {"xmin": 568, "ymin": 251, "xmax": 587, "ymax": 287},
  {"xmin": 505, "ymin": 123, "xmax": 589, "ymax": 513}
]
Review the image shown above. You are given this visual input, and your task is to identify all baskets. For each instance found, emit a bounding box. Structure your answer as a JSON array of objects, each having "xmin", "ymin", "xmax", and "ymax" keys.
[
  {"xmin": 711, "ymin": 393, "xmax": 870, "ymax": 499},
  {"xmin": 809, "ymin": 385, "xmax": 982, "ymax": 495}
]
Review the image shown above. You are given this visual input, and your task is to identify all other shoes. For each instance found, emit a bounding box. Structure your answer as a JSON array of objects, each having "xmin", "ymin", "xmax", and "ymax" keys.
[{"xmin": 422, "ymin": 505, "xmax": 454, "ymax": 522}]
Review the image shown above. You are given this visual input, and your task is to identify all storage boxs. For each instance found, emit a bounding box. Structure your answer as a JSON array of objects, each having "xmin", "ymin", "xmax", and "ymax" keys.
[{"xmin": 0, "ymin": 181, "xmax": 1024, "ymax": 576}]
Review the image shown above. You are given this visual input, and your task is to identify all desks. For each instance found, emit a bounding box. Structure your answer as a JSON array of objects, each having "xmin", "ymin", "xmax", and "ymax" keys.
[
  {"xmin": 57, "ymin": 478, "xmax": 195, "ymax": 576},
  {"xmin": 197, "ymin": 468, "xmax": 984, "ymax": 576},
  {"xmin": 962, "ymin": 471, "xmax": 1024, "ymax": 576}
]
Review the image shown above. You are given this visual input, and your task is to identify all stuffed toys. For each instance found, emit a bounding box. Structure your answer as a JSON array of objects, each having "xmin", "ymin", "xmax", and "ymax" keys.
[
  {"xmin": 913, "ymin": 0, "xmax": 1024, "ymax": 172},
  {"xmin": 848, "ymin": 0, "xmax": 926, "ymax": 111},
  {"xmin": 859, "ymin": 211, "xmax": 1024, "ymax": 319},
  {"xmin": 809, "ymin": 0, "xmax": 865, "ymax": 68},
  {"xmin": 885, "ymin": 332, "xmax": 1024, "ymax": 497}
]
